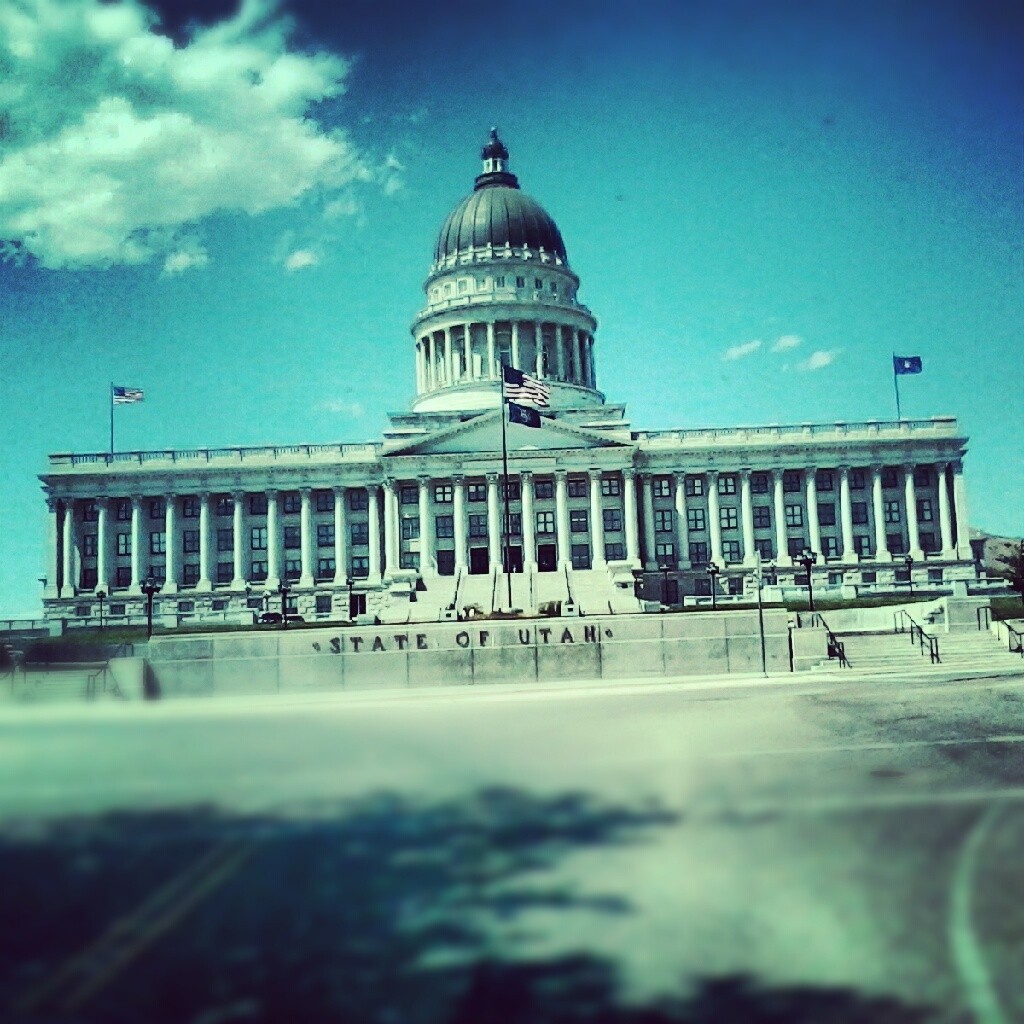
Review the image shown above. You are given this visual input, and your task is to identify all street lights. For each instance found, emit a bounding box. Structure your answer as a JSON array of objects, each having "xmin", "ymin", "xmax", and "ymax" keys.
[
  {"xmin": 280, "ymin": 582, "xmax": 291, "ymax": 626},
  {"xmin": 794, "ymin": 547, "xmax": 818, "ymax": 610},
  {"xmin": 141, "ymin": 578, "xmax": 162, "ymax": 638},
  {"xmin": 347, "ymin": 578, "xmax": 354, "ymax": 621},
  {"xmin": 706, "ymin": 562, "xmax": 720, "ymax": 609},
  {"xmin": 97, "ymin": 591, "xmax": 106, "ymax": 629},
  {"xmin": 905, "ymin": 555, "xmax": 914, "ymax": 593},
  {"xmin": 659, "ymin": 563, "xmax": 671, "ymax": 607}
]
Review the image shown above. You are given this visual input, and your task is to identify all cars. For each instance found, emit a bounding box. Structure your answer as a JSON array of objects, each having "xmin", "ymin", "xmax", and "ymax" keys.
[{"xmin": 259, "ymin": 614, "xmax": 305, "ymax": 623}]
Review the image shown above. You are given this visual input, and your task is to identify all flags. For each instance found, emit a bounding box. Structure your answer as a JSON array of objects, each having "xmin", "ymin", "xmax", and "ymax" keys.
[
  {"xmin": 508, "ymin": 402, "xmax": 541, "ymax": 428},
  {"xmin": 503, "ymin": 366, "xmax": 550, "ymax": 407},
  {"xmin": 894, "ymin": 357, "xmax": 922, "ymax": 376},
  {"xmin": 113, "ymin": 386, "xmax": 144, "ymax": 405}
]
